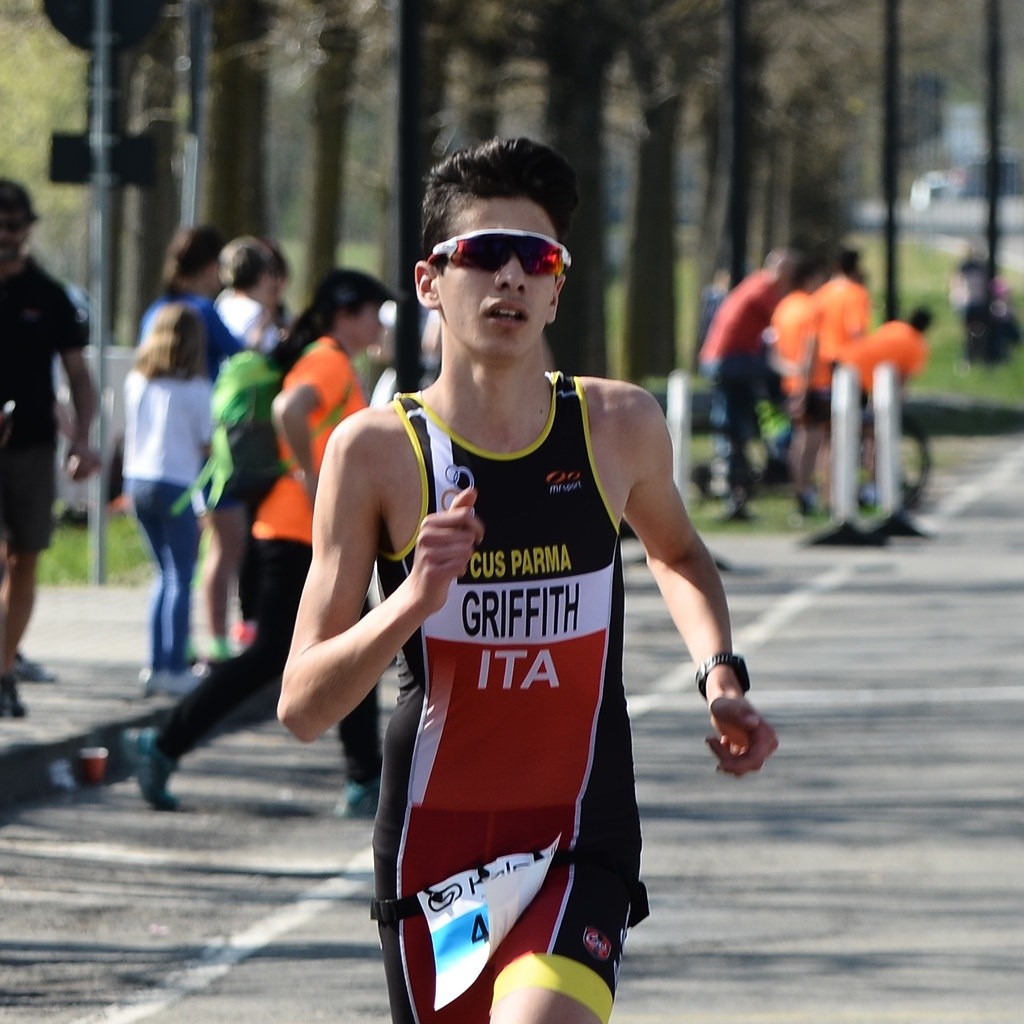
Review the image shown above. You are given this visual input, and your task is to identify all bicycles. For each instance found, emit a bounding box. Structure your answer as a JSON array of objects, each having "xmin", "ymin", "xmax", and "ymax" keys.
[{"xmin": 694, "ymin": 388, "xmax": 931, "ymax": 507}]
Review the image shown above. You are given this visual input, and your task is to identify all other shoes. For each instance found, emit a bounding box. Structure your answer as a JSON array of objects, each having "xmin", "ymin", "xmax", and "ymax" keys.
[
  {"xmin": 145, "ymin": 668, "xmax": 202, "ymax": 695},
  {"xmin": 2, "ymin": 677, "xmax": 23, "ymax": 713},
  {"xmin": 13, "ymin": 657, "xmax": 48, "ymax": 681}
]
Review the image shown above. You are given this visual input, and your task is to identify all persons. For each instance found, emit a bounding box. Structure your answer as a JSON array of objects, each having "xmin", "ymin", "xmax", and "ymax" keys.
[
  {"xmin": 0, "ymin": 175, "xmax": 98, "ymax": 716},
  {"xmin": 277, "ymin": 137, "xmax": 782, "ymax": 1024},
  {"xmin": 696, "ymin": 240, "xmax": 932, "ymax": 517},
  {"xmin": 114, "ymin": 219, "xmax": 391, "ymax": 820}
]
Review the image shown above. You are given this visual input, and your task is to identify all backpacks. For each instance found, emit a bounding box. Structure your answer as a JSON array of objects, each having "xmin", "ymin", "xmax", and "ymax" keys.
[{"xmin": 204, "ymin": 342, "xmax": 351, "ymax": 506}]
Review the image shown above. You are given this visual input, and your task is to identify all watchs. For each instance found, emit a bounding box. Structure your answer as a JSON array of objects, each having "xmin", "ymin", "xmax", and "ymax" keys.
[{"xmin": 694, "ymin": 653, "xmax": 751, "ymax": 700}]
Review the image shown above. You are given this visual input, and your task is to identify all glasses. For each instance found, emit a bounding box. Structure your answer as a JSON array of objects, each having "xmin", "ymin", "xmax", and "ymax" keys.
[
  {"xmin": 427, "ymin": 229, "xmax": 571, "ymax": 278},
  {"xmin": 0, "ymin": 219, "xmax": 28, "ymax": 234}
]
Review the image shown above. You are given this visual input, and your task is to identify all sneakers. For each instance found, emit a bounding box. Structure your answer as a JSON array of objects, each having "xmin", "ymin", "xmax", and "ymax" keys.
[
  {"xmin": 337, "ymin": 783, "xmax": 379, "ymax": 817},
  {"xmin": 125, "ymin": 727, "xmax": 178, "ymax": 810}
]
